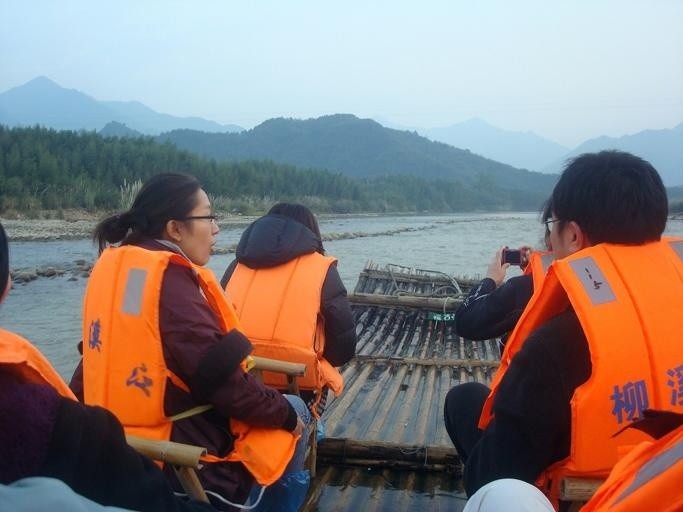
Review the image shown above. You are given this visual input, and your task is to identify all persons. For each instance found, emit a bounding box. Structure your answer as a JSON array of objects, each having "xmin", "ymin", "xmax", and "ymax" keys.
[
  {"xmin": 67, "ymin": 172, "xmax": 310, "ymax": 511},
  {"xmin": 0, "ymin": 221, "xmax": 174, "ymax": 511},
  {"xmin": 219, "ymin": 202, "xmax": 356, "ymax": 420}
]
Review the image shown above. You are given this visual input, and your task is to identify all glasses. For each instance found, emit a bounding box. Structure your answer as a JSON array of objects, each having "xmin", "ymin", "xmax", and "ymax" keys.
[
  {"xmin": 544, "ymin": 215, "xmax": 558, "ymax": 234},
  {"xmin": 170, "ymin": 212, "xmax": 219, "ymax": 224}
]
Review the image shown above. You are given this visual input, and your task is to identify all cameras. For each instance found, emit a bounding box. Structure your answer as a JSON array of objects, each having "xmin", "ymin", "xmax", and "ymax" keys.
[{"xmin": 504, "ymin": 248, "xmax": 524, "ymax": 265}]
